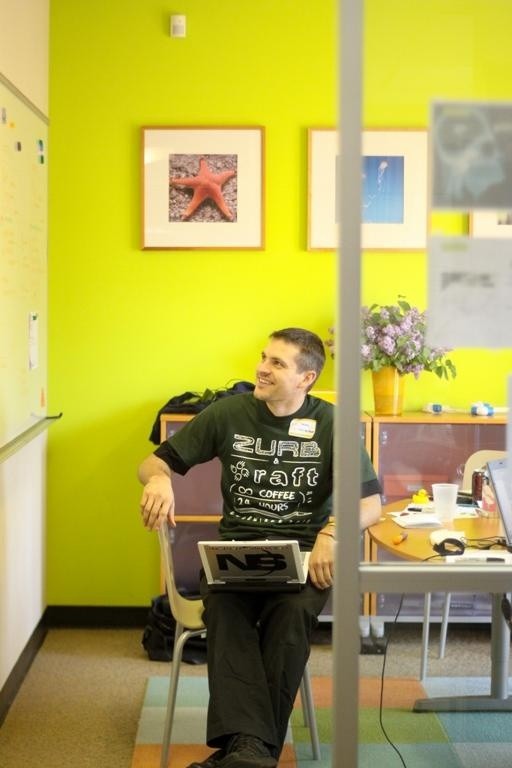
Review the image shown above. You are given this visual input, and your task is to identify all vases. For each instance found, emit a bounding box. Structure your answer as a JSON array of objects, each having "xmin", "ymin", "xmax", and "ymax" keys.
[{"xmin": 371, "ymin": 364, "xmax": 406, "ymax": 417}]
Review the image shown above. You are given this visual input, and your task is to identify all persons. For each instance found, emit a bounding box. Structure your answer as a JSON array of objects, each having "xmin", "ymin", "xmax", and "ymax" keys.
[{"xmin": 138, "ymin": 328, "xmax": 383, "ymax": 768}]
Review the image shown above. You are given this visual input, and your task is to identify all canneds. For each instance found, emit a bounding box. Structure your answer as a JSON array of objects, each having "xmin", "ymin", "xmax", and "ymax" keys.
[{"xmin": 472, "ymin": 470, "xmax": 490, "ymax": 504}]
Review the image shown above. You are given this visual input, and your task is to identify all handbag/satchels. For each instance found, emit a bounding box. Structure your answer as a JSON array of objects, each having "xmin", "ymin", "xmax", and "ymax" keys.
[{"xmin": 142, "ymin": 592, "xmax": 208, "ymax": 665}]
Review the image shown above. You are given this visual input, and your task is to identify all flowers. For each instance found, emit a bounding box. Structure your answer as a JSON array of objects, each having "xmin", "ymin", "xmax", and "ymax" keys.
[{"xmin": 324, "ymin": 297, "xmax": 459, "ymax": 383}]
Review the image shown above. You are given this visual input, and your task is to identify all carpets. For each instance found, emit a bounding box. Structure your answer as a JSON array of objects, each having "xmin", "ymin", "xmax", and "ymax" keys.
[{"xmin": 128, "ymin": 673, "xmax": 511, "ymax": 767}]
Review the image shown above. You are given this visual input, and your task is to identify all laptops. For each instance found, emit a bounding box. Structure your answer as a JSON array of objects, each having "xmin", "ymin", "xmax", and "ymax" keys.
[
  {"xmin": 197, "ymin": 539, "xmax": 312, "ymax": 593},
  {"xmin": 487, "ymin": 456, "xmax": 512, "ymax": 547}
]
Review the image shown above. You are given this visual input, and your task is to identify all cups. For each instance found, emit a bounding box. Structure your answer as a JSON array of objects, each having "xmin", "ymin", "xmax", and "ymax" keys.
[
  {"xmin": 480, "ymin": 474, "xmax": 498, "ymax": 519},
  {"xmin": 431, "ymin": 483, "xmax": 459, "ymax": 522}
]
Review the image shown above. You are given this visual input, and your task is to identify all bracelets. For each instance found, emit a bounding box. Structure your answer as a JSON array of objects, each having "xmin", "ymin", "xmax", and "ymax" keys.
[
  {"xmin": 327, "ymin": 518, "xmax": 334, "ymax": 527},
  {"xmin": 317, "ymin": 529, "xmax": 334, "ymax": 537}
]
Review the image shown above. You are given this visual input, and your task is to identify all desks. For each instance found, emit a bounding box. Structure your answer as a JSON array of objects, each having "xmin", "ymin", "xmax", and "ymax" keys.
[{"xmin": 368, "ymin": 493, "xmax": 511, "ymax": 712}]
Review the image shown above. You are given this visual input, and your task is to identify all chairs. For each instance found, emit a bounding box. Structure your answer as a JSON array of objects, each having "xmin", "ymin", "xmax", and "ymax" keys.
[
  {"xmin": 419, "ymin": 450, "xmax": 512, "ymax": 682},
  {"xmin": 157, "ymin": 507, "xmax": 321, "ymax": 767}
]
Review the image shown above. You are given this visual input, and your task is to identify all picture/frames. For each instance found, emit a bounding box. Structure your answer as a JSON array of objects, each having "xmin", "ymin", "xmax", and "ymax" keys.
[
  {"xmin": 305, "ymin": 126, "xmax": 431, "ymax": 254},
  {"xmin": 142, "ymin": 123, "xmax": 266, "ymax": 250}
]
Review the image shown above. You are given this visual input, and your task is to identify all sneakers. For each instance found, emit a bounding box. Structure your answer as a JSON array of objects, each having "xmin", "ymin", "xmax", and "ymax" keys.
[{"xmin": 188, "ymin": 734, "xmax": 280, "ymax": 767}]
